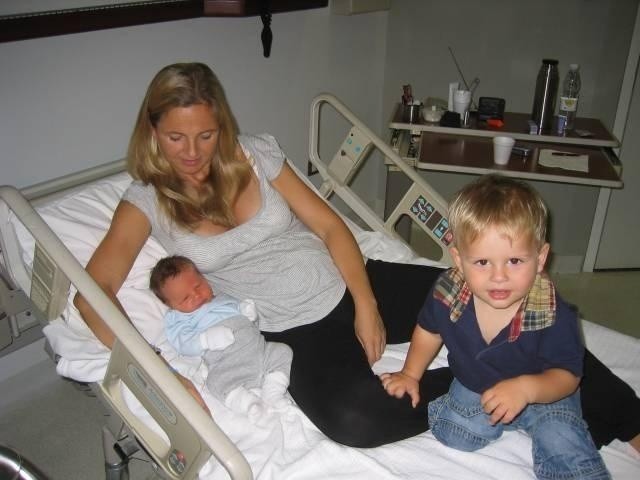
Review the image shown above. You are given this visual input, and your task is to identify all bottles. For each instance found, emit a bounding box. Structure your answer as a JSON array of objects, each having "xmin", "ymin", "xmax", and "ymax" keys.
[
  {"xmin": 532, "ymin": 58, "xmax": 560, "ymax": 130},
  {"xmin": 557, "ymin": 63, "xmax": 582, "ymax": 132}
]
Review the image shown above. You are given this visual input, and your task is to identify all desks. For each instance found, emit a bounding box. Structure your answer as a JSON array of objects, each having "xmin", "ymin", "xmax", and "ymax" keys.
[{"xmin": 385, "ymin": 99, "xmax": 622, "ymax": 278}]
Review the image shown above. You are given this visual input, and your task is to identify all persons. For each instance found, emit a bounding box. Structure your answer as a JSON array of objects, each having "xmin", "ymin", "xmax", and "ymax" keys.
[
  {"xmin": 148, "ymin": 254, "xmax": 295, "ymax": 424},
  {"xmin": 379, "ymin": 172, "xmax": 616, "ymax": 480},
  {"xmin": 70, "ymin": 59, "xmax": 638, "ymax": 453}
]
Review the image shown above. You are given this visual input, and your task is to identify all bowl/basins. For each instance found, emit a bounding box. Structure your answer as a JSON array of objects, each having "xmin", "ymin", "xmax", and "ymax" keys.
[{"xmin": 478, "ymin": 96, "xmax": 505, "ymax": 115}]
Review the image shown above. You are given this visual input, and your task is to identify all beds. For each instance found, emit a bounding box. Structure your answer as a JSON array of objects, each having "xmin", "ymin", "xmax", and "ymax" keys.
[{"xmin": 1, "ymin": 128, "xmax": 637, "ymax": 478}]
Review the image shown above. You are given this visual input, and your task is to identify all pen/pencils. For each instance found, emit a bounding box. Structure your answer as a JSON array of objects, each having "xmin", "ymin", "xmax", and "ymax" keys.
[{"xmin": 552, "ymin": 152, "xmax": 579, "ymax": 156}]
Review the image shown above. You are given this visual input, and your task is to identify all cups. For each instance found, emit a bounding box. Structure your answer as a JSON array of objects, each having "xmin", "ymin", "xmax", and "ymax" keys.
[
  {"xmin": 396, "ymin": 97, "xmax": 448, "ymax": 125},
  {"xmin": 492, "ymin": 136, "xmax": 515, "ymax": 166},
  {"xmin": 458, "ymin": 110, "xmax": 473, "ymax": 128},
  {"xmin": 454, "ymin": 89, "xmax": 473, "ymax": 115}
]
